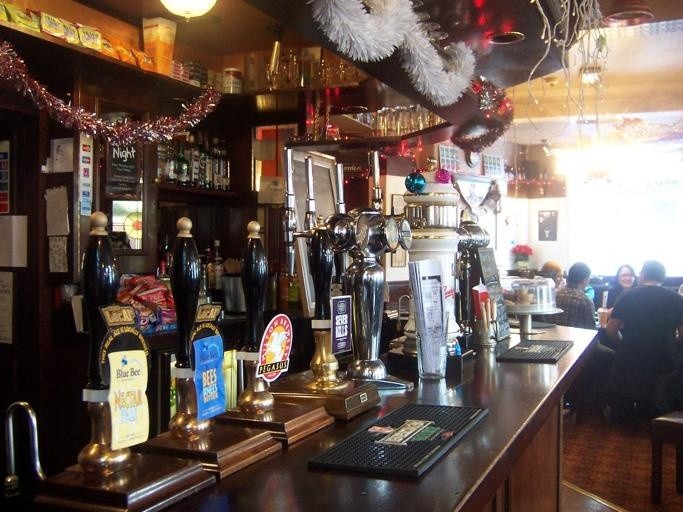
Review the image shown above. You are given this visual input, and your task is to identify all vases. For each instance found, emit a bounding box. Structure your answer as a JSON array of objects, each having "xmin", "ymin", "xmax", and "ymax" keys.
[{"xmin": 517, "ymin": 253, "xmax": 529, "ymax": 268}]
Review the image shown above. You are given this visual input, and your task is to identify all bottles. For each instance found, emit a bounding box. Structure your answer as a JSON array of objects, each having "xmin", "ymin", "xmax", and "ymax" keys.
[
  {"xmin": 210, "ymin": 240, "xmax": 223, "ymax": 297},
  {"xmin": 352, "ymin": 103, "xmax": 444, "ymax": 138},
  {"xmin": 156, "ymin": 129, "xmax": 231, "ymax": 190},
  {"xmin": 158, "ymin": 233, "xmax": 173, "ymax": 276},
  {"xmin": 201, "ymin": 246, "xmax": 215, "ymax": 297}
]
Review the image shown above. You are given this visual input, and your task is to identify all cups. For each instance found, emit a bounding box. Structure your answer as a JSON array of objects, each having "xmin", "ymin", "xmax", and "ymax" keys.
[
  {"xmin": 413, "ymin": 311, "xmax": 449, "ymax": 380},
  {"xmin": 597, "ymin": 307, "xmax": 609, "ymax": 328}
]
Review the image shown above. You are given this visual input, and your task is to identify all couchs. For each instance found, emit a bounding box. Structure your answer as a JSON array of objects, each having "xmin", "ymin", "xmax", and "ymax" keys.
[{"xmin": 591, "ymin": 275, "xmax": 683, "ymax": 312}]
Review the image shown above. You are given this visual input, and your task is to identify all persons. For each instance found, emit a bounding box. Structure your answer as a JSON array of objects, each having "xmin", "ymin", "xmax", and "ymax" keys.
[
  {"xmin": 534, "ymin": 261, "xmax": 683, "ymax": 422},
  {"xmin": 539, "ymin": 211, "xmax": 556, "ymax": 240}
]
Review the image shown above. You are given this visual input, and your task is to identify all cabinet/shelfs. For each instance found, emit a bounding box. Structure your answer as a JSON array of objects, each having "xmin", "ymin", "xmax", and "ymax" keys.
[{"xmin": 0, "ymin": 19, "xmax": 248, "ymax": 442}]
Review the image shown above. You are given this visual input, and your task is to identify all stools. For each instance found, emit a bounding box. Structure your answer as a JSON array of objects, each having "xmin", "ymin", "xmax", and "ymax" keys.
[{"xmin": 651, "ymin": 410, "xmax": 683, "ymax": 503}]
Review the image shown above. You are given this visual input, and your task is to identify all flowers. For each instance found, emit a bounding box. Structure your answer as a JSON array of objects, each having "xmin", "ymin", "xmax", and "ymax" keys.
[{"xmin": 512, "ymin": 244, "xmax": 534, "ymax": 262}]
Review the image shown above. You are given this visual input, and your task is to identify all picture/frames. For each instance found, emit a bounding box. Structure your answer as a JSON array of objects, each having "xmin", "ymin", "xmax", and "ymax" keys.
[{"xmin": 285, "ymin": 147, "xmax": 339, "ymax": 320}]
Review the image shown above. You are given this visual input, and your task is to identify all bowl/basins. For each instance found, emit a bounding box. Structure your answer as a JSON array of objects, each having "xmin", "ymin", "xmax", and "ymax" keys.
[{"xmin": 224, "ymin": 272, "xmax": 279, "ymax": 315}]
